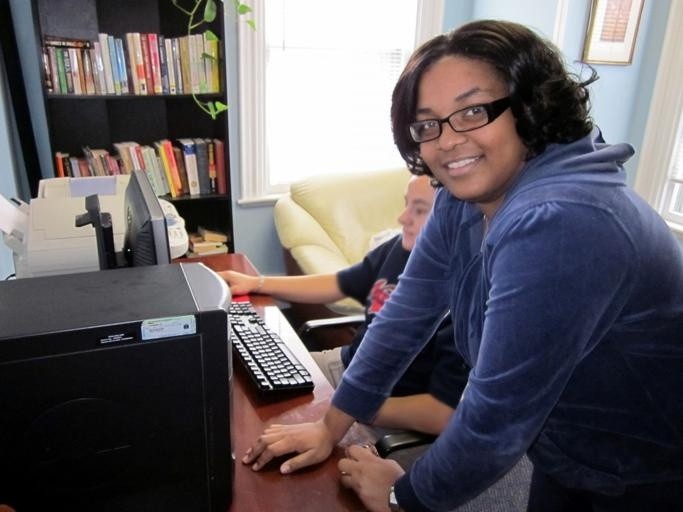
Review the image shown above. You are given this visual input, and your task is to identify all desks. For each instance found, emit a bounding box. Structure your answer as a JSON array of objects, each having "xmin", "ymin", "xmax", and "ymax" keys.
[{"xmin": 167, "ymin": 250, "xmax": 380, "ymax": 510}]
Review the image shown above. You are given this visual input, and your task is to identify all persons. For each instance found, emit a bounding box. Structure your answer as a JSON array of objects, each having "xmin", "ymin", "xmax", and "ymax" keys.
[
  {"xmin": 214, "ymin": 170, "xmax": 472, "ymax": 438},
  {"xmin": 239, "ymin": 18, "xmax": 681, "ymax": 511}
]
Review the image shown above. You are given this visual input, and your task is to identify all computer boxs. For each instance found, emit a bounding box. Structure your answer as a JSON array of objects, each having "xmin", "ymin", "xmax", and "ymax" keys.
[{"xmin": 0, "ymin": 261, "xmax": 235, "ymax": 512}]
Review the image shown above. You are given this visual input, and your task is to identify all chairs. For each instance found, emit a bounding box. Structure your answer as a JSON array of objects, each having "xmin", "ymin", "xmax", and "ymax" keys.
[{"xmin": 295, "ymin": 311, "xmax": 436, "ymax": 460}]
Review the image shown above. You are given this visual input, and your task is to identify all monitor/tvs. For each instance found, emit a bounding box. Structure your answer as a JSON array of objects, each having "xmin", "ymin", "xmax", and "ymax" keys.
[{"xmin": 72, "ymin": 170, "xmax": 171, "ymax": 271}]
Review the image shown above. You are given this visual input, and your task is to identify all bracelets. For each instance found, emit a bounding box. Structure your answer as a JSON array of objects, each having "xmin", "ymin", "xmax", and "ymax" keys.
[
  {"xmin": 254, "ymin": 276, "xmax": 265, "ymax": 293},
  {"xmin": 388, "ymin": 480, "xmax": 400, "ymax": 511}
]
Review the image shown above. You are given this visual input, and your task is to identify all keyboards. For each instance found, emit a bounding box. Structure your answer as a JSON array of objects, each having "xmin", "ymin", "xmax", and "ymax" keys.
[{"xmin": 229, "ymin": 302, "xmax": 315, "ymax": 408}]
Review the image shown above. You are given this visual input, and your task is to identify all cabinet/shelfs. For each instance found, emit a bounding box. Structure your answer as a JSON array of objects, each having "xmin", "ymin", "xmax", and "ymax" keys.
[{"xmin": 0, "ymin": 1, "xmax": 234, "ymax": 254}]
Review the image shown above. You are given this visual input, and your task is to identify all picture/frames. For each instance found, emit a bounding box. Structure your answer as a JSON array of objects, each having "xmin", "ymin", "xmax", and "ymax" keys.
[{"xmin": 580, "ymin": 0, "xmax": 645, "ymax": 67}]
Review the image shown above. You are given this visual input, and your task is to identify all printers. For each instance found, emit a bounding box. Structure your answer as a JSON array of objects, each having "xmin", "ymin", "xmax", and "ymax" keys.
[{"xmin": 0, "ymin": 174, "xmax": 190, "ymax": 279}]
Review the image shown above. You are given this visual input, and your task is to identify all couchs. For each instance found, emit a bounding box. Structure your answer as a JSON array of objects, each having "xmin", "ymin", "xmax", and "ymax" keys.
[{"xmin": 272, "ymin": 168, "xmax": 413, "ymax": 348}]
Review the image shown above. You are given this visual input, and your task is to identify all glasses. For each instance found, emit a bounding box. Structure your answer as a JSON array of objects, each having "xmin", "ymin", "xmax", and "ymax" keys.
[{"xmin": 410, "ymin": 94, "xmax": 514, "ymax": 143}]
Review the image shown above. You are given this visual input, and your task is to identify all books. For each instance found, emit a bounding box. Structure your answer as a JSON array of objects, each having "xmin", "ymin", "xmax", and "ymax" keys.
[
  {"xmin": 189, "ymin": 226, "xmax": 228, "ymax": 256},
  {"xmin": 39, "ymin": 30, "xmax": 220, "ymax": 96},
  {"xmin": 56, "ymin": 139, "xmax": 226, "ymax": 198}
]
